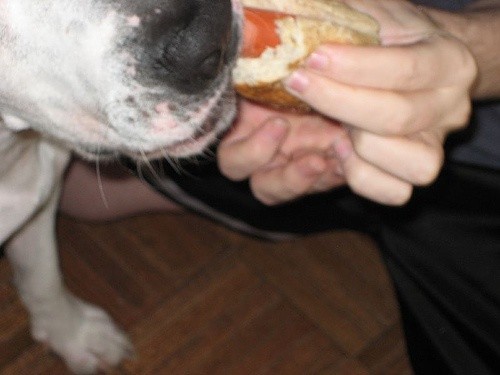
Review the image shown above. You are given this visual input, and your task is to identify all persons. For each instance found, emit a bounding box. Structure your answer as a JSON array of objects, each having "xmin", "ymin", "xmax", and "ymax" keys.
[{"xmin": 61, "ymin": 0, "xmax": 500, "ymax": 374}]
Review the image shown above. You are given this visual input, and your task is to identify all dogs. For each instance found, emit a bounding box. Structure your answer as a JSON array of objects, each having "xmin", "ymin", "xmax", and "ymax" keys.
[{"xmin": 1, "ymin": 0, "xmax": 242, "ymax": 375}]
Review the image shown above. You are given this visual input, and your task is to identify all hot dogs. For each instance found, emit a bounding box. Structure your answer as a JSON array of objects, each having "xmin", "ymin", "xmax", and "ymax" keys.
[{"xmin": 231, "ymin": 0, "xmax": 381, "ymax": 111}]
